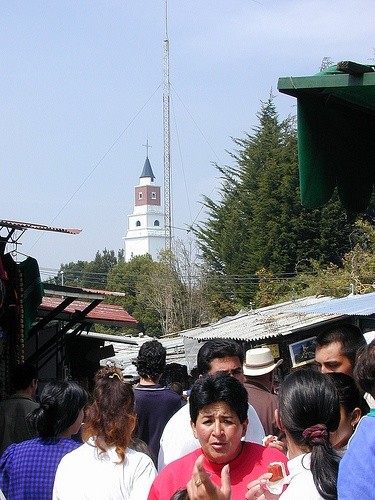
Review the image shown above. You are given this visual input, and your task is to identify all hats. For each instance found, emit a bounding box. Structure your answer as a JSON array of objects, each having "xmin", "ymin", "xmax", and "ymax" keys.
[{"xmin": 243, "ymin": 348, "xmax": 283, "ymax": 376}]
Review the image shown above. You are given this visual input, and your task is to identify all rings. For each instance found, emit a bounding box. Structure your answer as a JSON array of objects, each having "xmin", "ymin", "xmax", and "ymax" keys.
[{"xmin": 195, "ymin": 479, "xmax": 202, "ymax": 487}]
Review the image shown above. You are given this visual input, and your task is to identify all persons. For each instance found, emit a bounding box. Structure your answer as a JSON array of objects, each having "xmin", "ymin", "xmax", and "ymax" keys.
[
  {"xmin": 246, "ymin": 368, "xmax": 341, "ymax": 500},
  {"xmin": 147, "ymin": 371, "xmax": 288, "ymax": 500},
  {"xmin": 0, "ymin": 324, "xmax": 366, "ymax": 474},
  {"xmin": 338, "ymin": 339, "xmax": 375, "ymax": 500},
  {"xmin": 52, "ymin": 367, "xmax": 158, "ymax": 500},
  {"xmin": 0, "ymin": 381, "xmax": 88, "ymax": 500}
]
